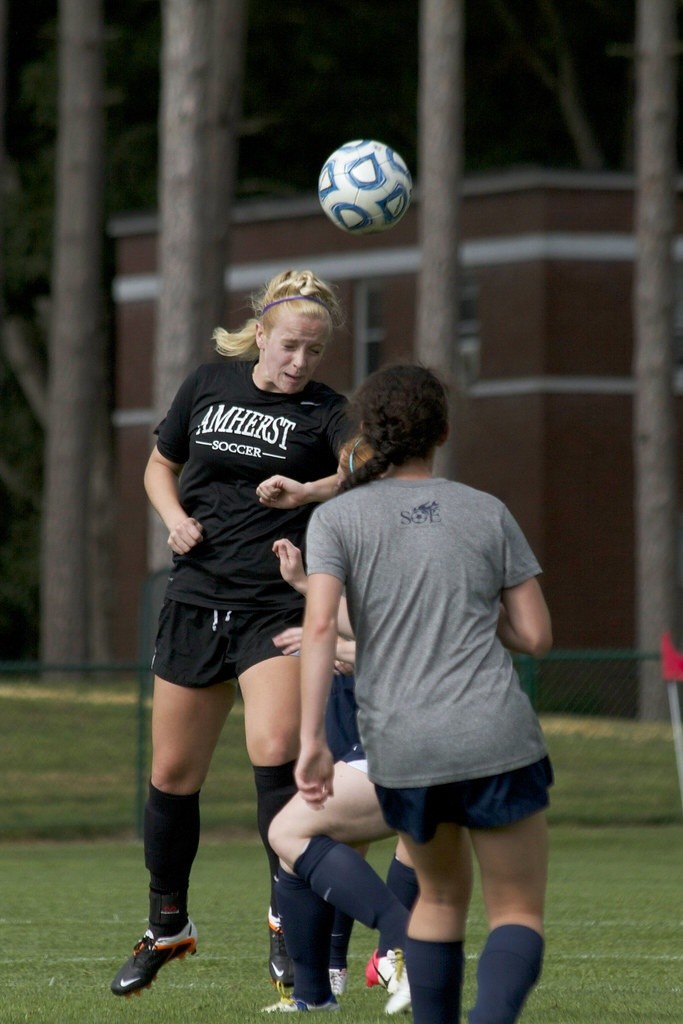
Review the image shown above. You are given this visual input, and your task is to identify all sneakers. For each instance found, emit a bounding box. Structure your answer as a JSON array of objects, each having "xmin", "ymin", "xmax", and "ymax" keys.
[
  {"xmin": 107, "ymin": 915, "xmax": 196, "ymax": 998},
  {"xmin": 384, "ymin": 991, "xmax": 413, "ymax": 1014},
  {"xmin": 259, "ymin": 994, "xmax": 339, "ymax": 1013},
  {"xmin": 267, "ymin": 906, "xmax": 295, "ymax": 989},
  {"xmin": 366, "ymin": 948, "xmax": 410, "ymax": 993},
  {"xmin": 329, "ymin": 969, "xmax": 351, "ymax": 1000}
]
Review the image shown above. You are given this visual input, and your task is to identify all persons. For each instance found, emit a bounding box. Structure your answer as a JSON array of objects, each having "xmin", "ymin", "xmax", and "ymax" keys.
[
  {"xmin": 111, "ymin": 268, "xmax": 364, "ymax": 1001},
  {"xmin": 295, "ymin": 364, "xmax": 557, "ymax": 1024},
  {"xmin": 255, "ymin": 441, "xmax": 428, "ymax": 1011}
]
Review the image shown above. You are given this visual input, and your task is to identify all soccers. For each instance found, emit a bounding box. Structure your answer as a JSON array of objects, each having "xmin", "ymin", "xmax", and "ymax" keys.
[{"xmin": 317, "ymin": 140, "xmax": 413, "ymax": 235}]
